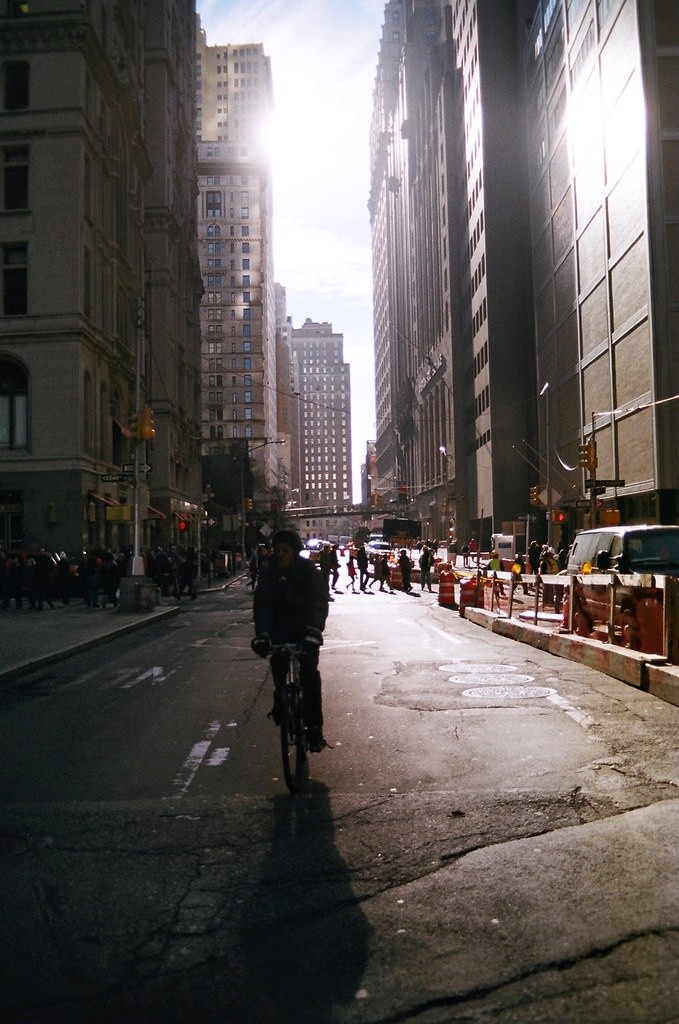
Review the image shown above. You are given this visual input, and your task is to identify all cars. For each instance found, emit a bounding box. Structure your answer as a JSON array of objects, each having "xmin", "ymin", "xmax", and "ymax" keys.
[
  {"xmin": 9, "ymin": 551, "xmax": 60, "ymax": 575},
  {"xmin": 306, "ymin": 540, "xmax": 335, "ymax": 550},
  {"xmin": 365, "ymin": 541, "xmax": 395, "ymax": 561},
  {"xmin": 349, "ymin": 543, "xmax": 368, "ymax": 560}
]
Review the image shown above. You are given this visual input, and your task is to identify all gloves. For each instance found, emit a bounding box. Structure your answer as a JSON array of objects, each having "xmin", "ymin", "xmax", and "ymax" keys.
[
  {"xmin": 305, "ymin": 626, "xmax": 324, "ymax": 646},
  {"xmin": 251, "ymin": 633, "xmax": 272, "ymax": 658}
]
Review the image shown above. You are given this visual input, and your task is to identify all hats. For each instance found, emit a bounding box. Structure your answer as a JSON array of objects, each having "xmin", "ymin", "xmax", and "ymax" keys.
[
  {"xmin": 423, "ymin": 547, "xmax": 428, "ymax": 550},
  {"xmin": 492, "ymin": 549, "xmax": 498, "ymax": 555}
]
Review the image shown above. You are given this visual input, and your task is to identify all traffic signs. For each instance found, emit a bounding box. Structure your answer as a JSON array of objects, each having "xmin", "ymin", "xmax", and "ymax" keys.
[
  {"xmin": 201, "ymin": 517, "xmax": 217, "ymax": 526},
  {"xmin": 518, "ymin": 514, "xmax": 539, "ymax": 522},
  {"xmin": 123, "ymin": 463, "xmax": 154, "ymax": 474}
]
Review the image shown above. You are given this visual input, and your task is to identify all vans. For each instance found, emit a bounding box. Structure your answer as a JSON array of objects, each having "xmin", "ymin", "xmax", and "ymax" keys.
[{"xmin": 566, "ymin": 524, "xmax": 679, "ymax": 652}]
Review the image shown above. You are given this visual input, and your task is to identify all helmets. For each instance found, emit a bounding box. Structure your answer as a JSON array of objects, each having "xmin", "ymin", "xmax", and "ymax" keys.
[{"xmin": 272, "ymin": 528, "xmax": 302, "ymax": 551}]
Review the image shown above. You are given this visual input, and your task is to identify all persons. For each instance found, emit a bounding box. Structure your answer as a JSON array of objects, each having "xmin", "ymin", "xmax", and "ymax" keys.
[
  {"xmin": 253, "ymin": 529, "xmax": 329, "ymax": 752},
  {"xmin": 0, "ymin": 545, "xmax": 271, "ymax": 609},
  {"xmin": 317, "ymin": 537, "xmax": 565, "ymax": 614}
]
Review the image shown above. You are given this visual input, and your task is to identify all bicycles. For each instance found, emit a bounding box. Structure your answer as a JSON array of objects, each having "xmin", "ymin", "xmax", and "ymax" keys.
[{"xmin": 260, "ymin": 643, "xmax": 327, "ymax": 795}]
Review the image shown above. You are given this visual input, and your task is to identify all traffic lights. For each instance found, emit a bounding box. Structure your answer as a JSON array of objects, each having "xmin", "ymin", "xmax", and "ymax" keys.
[
  {"xmin": 556, "ymin": 511, "xmax": 567, "ymax": 523},
  {"xmin": 377, "ymin": 494, "xmax": 382, "ymax": 505},
  {"xmin": 143, "ymin": 406, "xmax": 156, "ymax": 439},
  {"xmin": 529, "ymin": 487, "xmax": 539, "ymax": 505},
  {"xmin": 130, "ymin": 412, "xmax": 143, "ymax": 441},
  {"xmin": 578, "ymin": 441, "xmax": 596, "ymax": 470},
  {"xmin": 247, "ymin": 498, "xmax": 252, "ymax": 509},
  {"xmin": 179, "ymin": 520, "xmax": 190, "ymax": 531},
  {"xmin": 371, "ymin": 494, "xmax": 375, "ymax": 504}
]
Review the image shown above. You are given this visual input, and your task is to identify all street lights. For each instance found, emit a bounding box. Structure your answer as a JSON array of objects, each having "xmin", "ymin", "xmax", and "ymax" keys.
[
  {"xmin": 367, "ymin": 475, "xmax": 398, "ymax": 519},
  {"xmin": 240, "ymin": 440, "xmax": 286, "ymax": 569}
]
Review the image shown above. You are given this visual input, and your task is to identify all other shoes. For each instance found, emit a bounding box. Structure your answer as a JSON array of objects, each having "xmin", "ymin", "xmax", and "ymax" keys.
[{"xmin": 307, "ymin": 725, "xmax": 322, "ymax": 752}]
